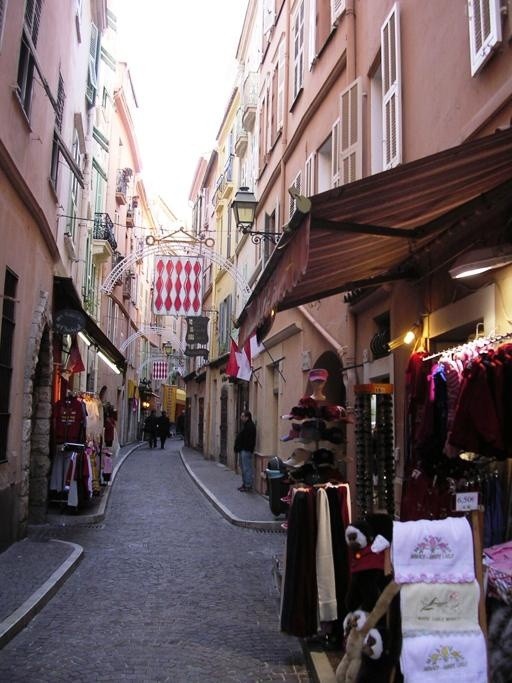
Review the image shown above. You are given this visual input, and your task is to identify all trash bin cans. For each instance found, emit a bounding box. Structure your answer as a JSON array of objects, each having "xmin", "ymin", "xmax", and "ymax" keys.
[{"xmin": 265, "ymin": 455, "xmax": 288, "ymax": 517}]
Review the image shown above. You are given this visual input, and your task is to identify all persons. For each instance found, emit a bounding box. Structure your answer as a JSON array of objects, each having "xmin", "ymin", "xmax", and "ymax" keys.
[
  {"xmin": 177, "ymin": 409, "xmax": 186, "ymax": 439},
  {"xmin": 158, "ymin": 411, "xmax": 170, "ymax": 449},
  {"xmin": 144, "ymin": 410, "xmax": 160, "ymax": 448},
  {"xmin": 234, "ymin": 411, "xmax": 256, "ymax": 491}
]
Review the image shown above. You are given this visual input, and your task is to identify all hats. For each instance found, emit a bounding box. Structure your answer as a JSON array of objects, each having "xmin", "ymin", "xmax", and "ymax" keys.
[
  {"xmin": 280, "ymin": 406, "xmax": 353, "ymax": 444},
  {"xmin": 308, "ymin": 368, "xmax": 328, "ymax": 382},
  {"xmin": 282, "ymin": 448, "xmax": 345, "ymax": 482}
]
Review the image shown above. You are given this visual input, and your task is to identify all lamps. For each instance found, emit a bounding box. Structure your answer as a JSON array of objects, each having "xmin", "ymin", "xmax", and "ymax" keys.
[{"xmin": 228, "ymin": 183, "xmax": 286, "ymax": 245}]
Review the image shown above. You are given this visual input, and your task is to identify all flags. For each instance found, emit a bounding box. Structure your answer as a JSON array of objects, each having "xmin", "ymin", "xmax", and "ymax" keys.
[
  {"xmin": 241, "ymin": 328, "xmax": 265, "ymax": 372},
  {"xmin": 225, "ymin": 340, "xmax": 252, "ymax": 382}
]
